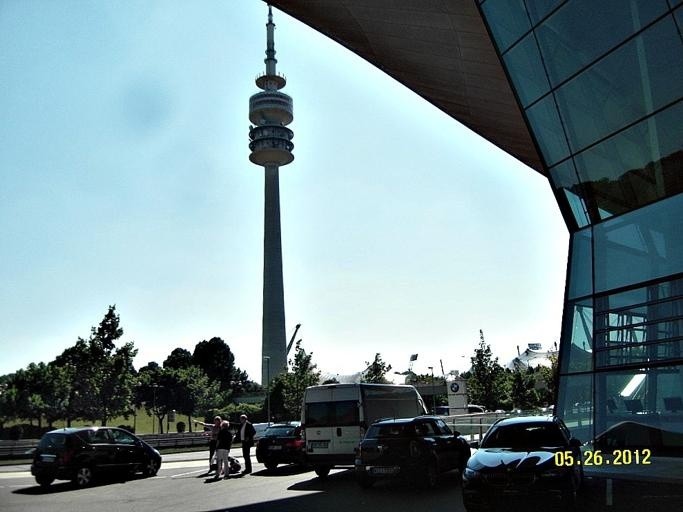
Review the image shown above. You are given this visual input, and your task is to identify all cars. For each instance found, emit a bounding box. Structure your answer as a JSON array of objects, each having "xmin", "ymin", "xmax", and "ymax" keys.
[
  {"xmin": 354, "ymin": 417, "xmax": 471, "ymax": 489},
  {"xmin": 462, "ymin": 415, "xmax": 584, "ymax": 510},
  {"xmin": 30, "ymin": 426, "xmax": 163, "ymax": 487},
  {"xmin": 255, "ymin": 425, "xmax": 306, "ymax": 470}
]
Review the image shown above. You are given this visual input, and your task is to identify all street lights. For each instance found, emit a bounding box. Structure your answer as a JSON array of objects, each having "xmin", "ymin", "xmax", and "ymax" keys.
[
  {"xmin": 264, "ymin": 355, "xmax": 270, "ymax": 425},
  {"xmin": 148, "ymin": 383, "xmax": 165, "ymax": 434}
]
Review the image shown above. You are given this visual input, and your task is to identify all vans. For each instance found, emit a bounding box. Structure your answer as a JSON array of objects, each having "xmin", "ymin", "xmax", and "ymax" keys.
[{"xmin": 300, "ymin": 383, "xmax": 430, "ymax": 477}]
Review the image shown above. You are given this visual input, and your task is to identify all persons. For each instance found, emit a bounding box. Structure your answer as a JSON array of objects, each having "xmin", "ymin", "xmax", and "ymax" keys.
[
  {"xmin": 236, "ymin": 414, "xmax": 256, "ymax": 474},
  {"xmin": 193, "ymin": 416, "xmax": 233, "ymax": 479}
]
